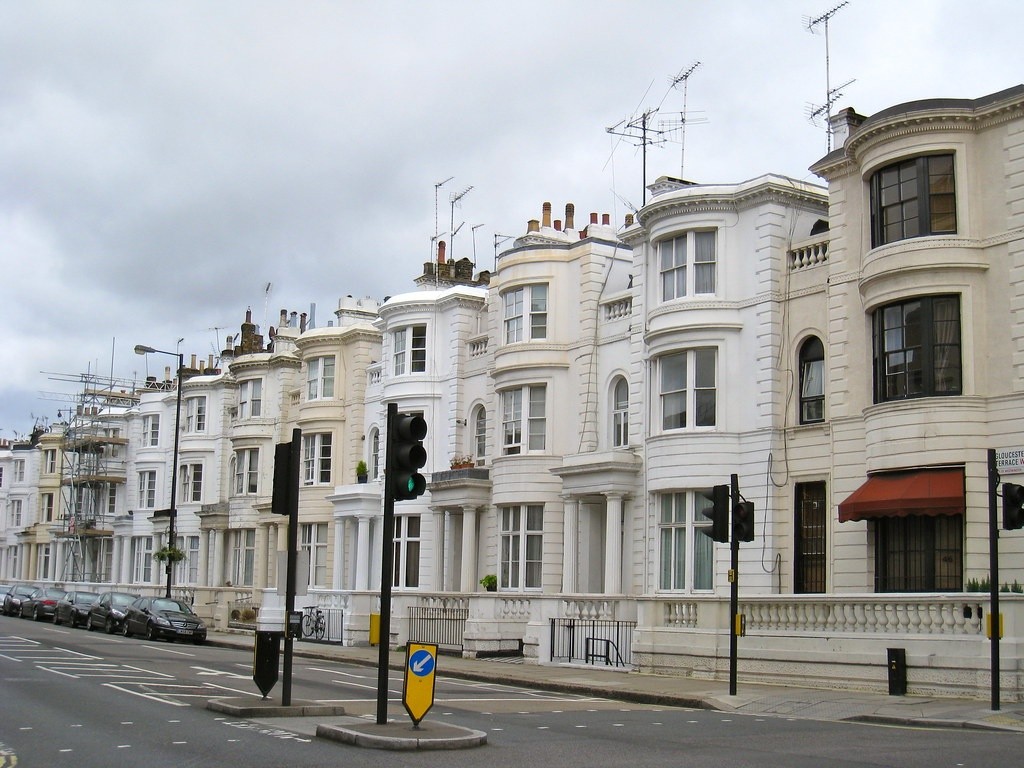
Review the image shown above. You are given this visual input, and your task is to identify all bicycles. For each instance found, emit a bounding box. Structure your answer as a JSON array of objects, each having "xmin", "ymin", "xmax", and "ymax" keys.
[{"xmin": 300, "ymin": 606, "xmax": 326, "ymax": 641}]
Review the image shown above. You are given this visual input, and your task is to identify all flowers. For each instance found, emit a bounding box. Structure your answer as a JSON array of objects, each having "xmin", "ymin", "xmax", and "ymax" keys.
[
  {"xmin": 461, "ymin": 453, "xmax": 475, "ymax": 464},
  {"xmin": 448, "ymin": 455, "xmax": 462, "ymax": 465}
]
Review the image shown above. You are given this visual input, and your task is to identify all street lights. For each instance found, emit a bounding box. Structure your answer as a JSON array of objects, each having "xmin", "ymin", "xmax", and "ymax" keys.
[{"xmin": 133, "ymin": 343, "xmax": 184, "ymax": 599}]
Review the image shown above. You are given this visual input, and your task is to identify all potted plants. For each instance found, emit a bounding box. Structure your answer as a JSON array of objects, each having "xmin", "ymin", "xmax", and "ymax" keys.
[
  {"xmin": 355, "ymin": 460, "xmax": 370, "ymax": 484},
  {"xmin": 479, "ymin": 574, "xmax": 497, "ymax": 592}
]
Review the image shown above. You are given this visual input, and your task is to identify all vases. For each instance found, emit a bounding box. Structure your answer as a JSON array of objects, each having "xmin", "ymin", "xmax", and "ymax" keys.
[
  {"xmin": 460, "ymin": 462, "xmax": 474, "ymax": 469},
  {"xmin": 450, "ymin": 464, "xmax": 462, "ymax": 470}
]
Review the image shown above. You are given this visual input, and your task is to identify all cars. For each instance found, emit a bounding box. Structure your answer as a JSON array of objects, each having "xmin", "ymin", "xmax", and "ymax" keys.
[
  {"xmin": 18, "ymin": 588, "xmax": 68, "ymax": 622},
  {"xmin": 52, "ymin": 591, "xmax": 100, "ymax": 628},
  {"xmin": 3, "ymin": 584, "xmax": 42, "ymax": 617},
  {"xmin": 86, "ymin": 591, "xmax": 140, "ymax": 633},
  {"xmin": 0, "ymin": 585, "xmax": 14, "ymax": 613},
  {"xmin": 121, "ymin": 596, "xmax": 207, "ymax": 646}
]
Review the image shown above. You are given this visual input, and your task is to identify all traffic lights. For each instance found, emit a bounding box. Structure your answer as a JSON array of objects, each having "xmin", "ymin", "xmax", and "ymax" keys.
[
  {"xmin": 389, "ymin": 412, "xmax": 428, "ymax": 500},
  {"xmin": 1001, "ymin": 481, "xmax": 1024, "ymax": 530},
  {"xmin": 701, "ymin": 486, "xmax": 729, "ymax": 543},
  {"xmin": 270, "ymin": 443, "xmax": 289, "ymax": 515},
  {"xmin": 734, "ymin": 501, "xmax": 755, "ymax": 543}
]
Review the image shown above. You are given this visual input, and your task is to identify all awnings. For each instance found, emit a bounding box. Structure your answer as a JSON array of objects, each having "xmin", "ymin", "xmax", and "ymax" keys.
[{"xmin": 837, "ymin": 471, "xmax": 964, "ymax": 523}]
